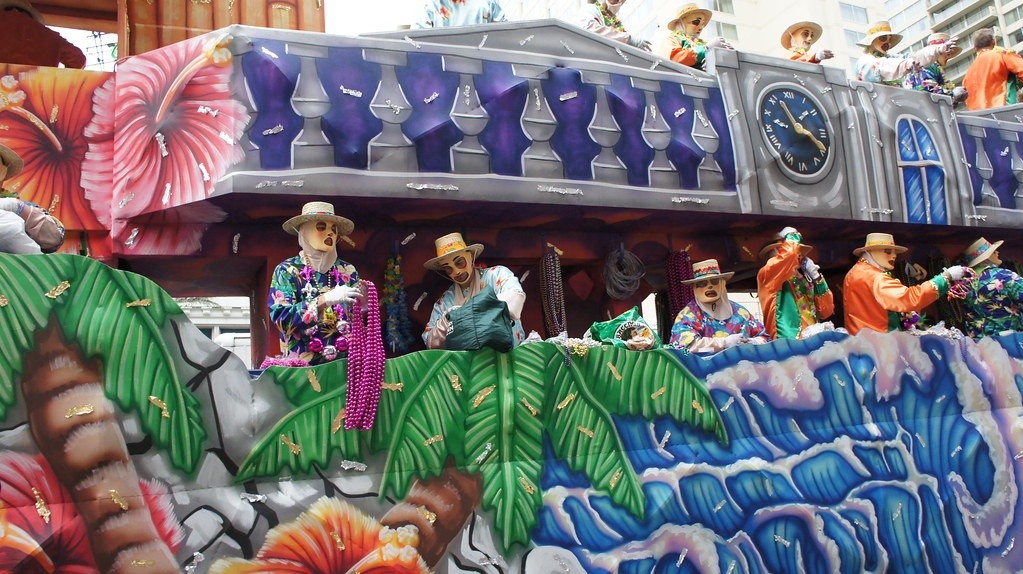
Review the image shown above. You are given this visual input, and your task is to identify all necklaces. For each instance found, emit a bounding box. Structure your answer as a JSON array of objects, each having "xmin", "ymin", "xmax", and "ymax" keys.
[
  {"xmin": 675, "ymin": 32, "xmax": 707, "ymax": 70},
  {"xmin": 540, "ymin": 253, "xmax": 586, "ymax": 366},
  {"xmin": 792, "ymin": 275, "xmax": 818, "ymax": 319},
  {"xmin": 305, "ymin": 253, "xmax": 335, "ymax": 325},
  {"xmin": 883, "ymin": 269, "xmax": 926, "ymax": 331},
  {"xmin": 344, "ymin": 279, "xmax": 385, "ymax": 433},
  {"xmin": 916, "ymin": 65, "xmax": 955, "ymax": 97},
  {"xmin": 668, "ymin": 250, "xmax": 694, "ymax": 326},
  {"xmin": 788, "ymin": 47, "xmax": 808, "ymax": 56},
  {"xmin": 594, "ymin": 2, "xmax": 626, "ymax": 32},
  {"xmin": 381, "ymin": 255, "xmax": 415, "ymax": 353}
]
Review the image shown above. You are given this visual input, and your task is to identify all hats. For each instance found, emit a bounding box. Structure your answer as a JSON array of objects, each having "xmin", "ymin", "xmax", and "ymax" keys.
[
  {"xmin": 666, "ymin": 3, "xmax": 712, "ymax": 29},
  {"xmin": 758, "ymin": 237, "xmax": 813, "ymax": 264},
  {"xmin": 852, "ymin": 233, "xmax": 908, "ymax": 258},
  {"xmin": 855, "ymin": 21, "xmax": 903, "ymax": 50},
  {"xmin": 961, "ymin": 236, "xmax": 1005, "ymax": 268},
  {"xmin": 1, "ymin": 145, "xmax": 22, "ymax": 181},
  {"xmin": 780, "ymin": 21, "xmax": 823, "ymax": 51},
  {"xmin": 927, "ymin": 33, "xmax": 962, "ymax": 60},
  {"xmin": 421, "ymin": 232, "xmax": 484, "ymax": 271},
  {"xmin": 680, "ymin": 259, "xmax": 735, "ymax": 287},
  {"xmin": 282, "ymin": 201, "xmax": 355, "ymax": 236}
]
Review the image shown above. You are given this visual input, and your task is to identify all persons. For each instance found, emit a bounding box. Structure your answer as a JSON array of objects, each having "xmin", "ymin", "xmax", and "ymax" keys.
[
  {"xmin": 410, "ymin": 0, "xmax": 509, "ymax": 30},
  {"xmin": 854, "ymin": 21, "xmax": 960, "ymax": 88},
  {"xmin": 843, "ymin": 232, "xmax": 965, "ymax": 337},
  {"xmin": 268, "ymin": 201, "xmax": 378, "ymax": 368},
  {"xmin": 780, "ymin": 21, "xmax": 834, "ymax": 64},
  {"xmin": 0, "ymin": 143, "xmax": 64, "ymax": 254},
  {"xmin": 961, "ymin": 28, "xmax": 1023, "ymax": 111},
  {"xmin": 421, "ymin": 232, "xmax": 526, "ymax": 350},
  {"xmin": 578, "ymin": 0, "xmax": 653, "ymax": 54},
  {"xmin": 959, "ymin": 236, "xmax": 1023, "ymax": 343},
  {"xmin": 903, "ymin": 33, "xmax": 968, "ymax": 109},
  {"xmin": 757, "ymin": 227, "xmax": 833, "ymax": 341},
  {"xmin": 669, "ymin": 258, "xmax": 768, "ymax": 358},
  {"xmin": 659, "ymin": 0, "xmax": 735, "ymax": 72}
]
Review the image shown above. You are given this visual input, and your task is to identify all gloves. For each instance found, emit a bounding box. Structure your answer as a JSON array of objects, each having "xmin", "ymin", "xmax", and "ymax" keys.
[
  {"xmin": 815, "ymin": 49, "xmax": 834, "ymax": 61},
  {"xmin": 725, "ymin": 333, "xmax": 746, "ymax": 349},
  {"xmin": 356, "ymin": 279, "xmax": 370, "ymax": 304},
  {"xmin": 747, "ymin": 337, "xmax": 767, "ymax": 346},
  {"xmin": 906, "ymin": 59, "xmax": 924, "ymax": 78},
  {"xmin": 913, "ymin": 263, "xmax": 927, "ymax": 280},
  {"xmin": 940, "ymin": 36, "xmax": 958, "ymax": 53},
  {"xmin": 952, "ymin": 86, "xmax": 968, "ymax": 102},
  {"xmin": 777, "ymin": 226, "xmax": 802, "ymax": 239},
  {"xmin": 801, "ymin": 256, "xmax": 820, "ymax": 280},
  {"xmin": 725, "ymin": 41, "xmax": 735, "ymax": 49},
  {"xmin": 628, "ymin": 34, "xmax": 653, "ymax": 53},
  {"xmin": 323, "ymin": 284, "xmax": 364, "ymax": 307},
  {"xmin": 943, "ymin": 264, "xmax": 978, "ymax": 281},
  {"xmin": 706, "ymin": 36, "xmax": 726, "ymax": 49}
]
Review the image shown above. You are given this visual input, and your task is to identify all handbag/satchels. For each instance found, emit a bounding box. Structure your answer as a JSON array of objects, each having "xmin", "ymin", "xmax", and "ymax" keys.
[{"xmin": 445, "ymin": 290, "xmax": 514, "ymax": 352}]
